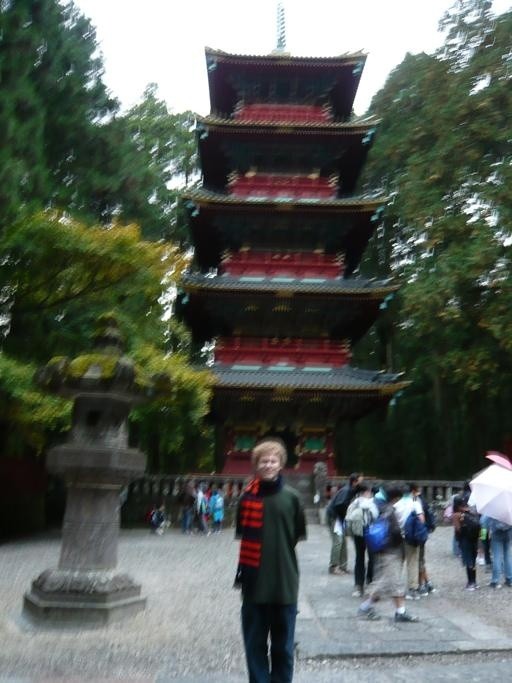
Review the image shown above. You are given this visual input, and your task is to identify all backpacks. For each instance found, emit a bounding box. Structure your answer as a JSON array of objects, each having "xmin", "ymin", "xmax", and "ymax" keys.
[
  {"xmin": 363, "ymin": 503, "xmax": 403, "ymax": 553},
  {"xmin": 343, "ymin": 498, "xmax": 365, "ymax": 537},
  {"xmin": 404, "ymin": 510, "xmax": 428, "ymax": 547}
]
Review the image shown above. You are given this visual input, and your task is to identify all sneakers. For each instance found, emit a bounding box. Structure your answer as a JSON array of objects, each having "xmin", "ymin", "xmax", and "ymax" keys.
[
  {"xmin": 464, "ymin": 579, "xmax": 512, "ymax": 591},
  {"xmin": 404, "ymin": 580, "xmax": 435, "ymax": 600},
  {"xmin": 357, "ymin": 605, "xmax": 381, "ymax": 621},
  {"xmin": 395, "ymin": 612, "xmax": 419, "ymax": 622},
  {"xmin": 329, "ymin": 564, "xmax": 372, "ymax": 598}
]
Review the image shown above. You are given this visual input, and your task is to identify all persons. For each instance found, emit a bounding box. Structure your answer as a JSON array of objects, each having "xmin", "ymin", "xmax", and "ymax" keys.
[
  {"xmin": 444, "ymin": 479, "xmax": 512, "ymax": 590},
  {"xmin": 182, "ymin": 480, "xmax": 245, "ymax": 536},
  {"xmin": 149, "ymin": 500, "xmax": 172, "ymax": 536},
  {"xmin": 231, "ymin": 439, "xmax": 307, "ymax": 683},
  {"xmin": 325, "ymin": 473, "xmax": 436, "ymax": 623}
]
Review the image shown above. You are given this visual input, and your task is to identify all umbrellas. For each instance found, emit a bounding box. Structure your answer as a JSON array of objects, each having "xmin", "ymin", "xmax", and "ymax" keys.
[{"xmin": 468, "ymin": 451, "xmax": 512, "ymax": 527}]
